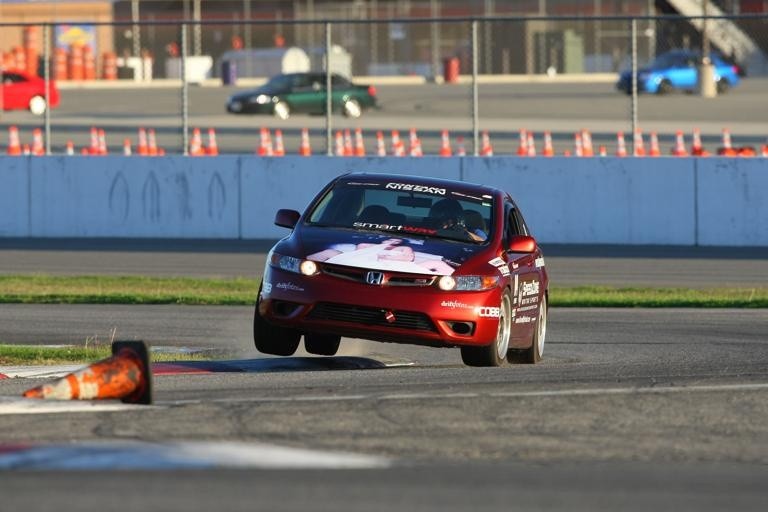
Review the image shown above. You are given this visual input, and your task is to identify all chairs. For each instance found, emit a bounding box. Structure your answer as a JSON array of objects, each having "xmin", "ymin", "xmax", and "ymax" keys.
[
  {"xmin": 463, "ymin": 210, "xmax": 486, "ymax": 232},
  {"xmin": 359, "ymin": 204, "xmax": 405, "ymax": 224}
]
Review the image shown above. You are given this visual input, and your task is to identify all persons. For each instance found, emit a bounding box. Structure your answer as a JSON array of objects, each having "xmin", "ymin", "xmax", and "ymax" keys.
[{"xmin": 427, "ymin": 198, "xmax": 488, "ymax": 244}]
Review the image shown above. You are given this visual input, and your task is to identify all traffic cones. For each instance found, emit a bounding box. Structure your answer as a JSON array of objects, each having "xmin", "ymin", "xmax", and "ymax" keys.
[{"xmin": 22, "ymin": 339, "xmax": 155, "ymax": 406}]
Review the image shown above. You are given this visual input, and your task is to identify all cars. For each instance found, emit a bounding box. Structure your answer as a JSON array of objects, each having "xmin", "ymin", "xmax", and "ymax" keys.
[
  {"xmin": 252, "ymin": 168, "xmax": 550, "ymax": 370},
  {"xmin": 225, "ymin": 70, "xmax": 379, "ymax": 122},
  {"xmin": 614, "ymin": 47, "xmax": 740, "ymax": 97},
  {"xmin": 0, "ymin": 67, "xmax": 62, "ymax": 116}
]
